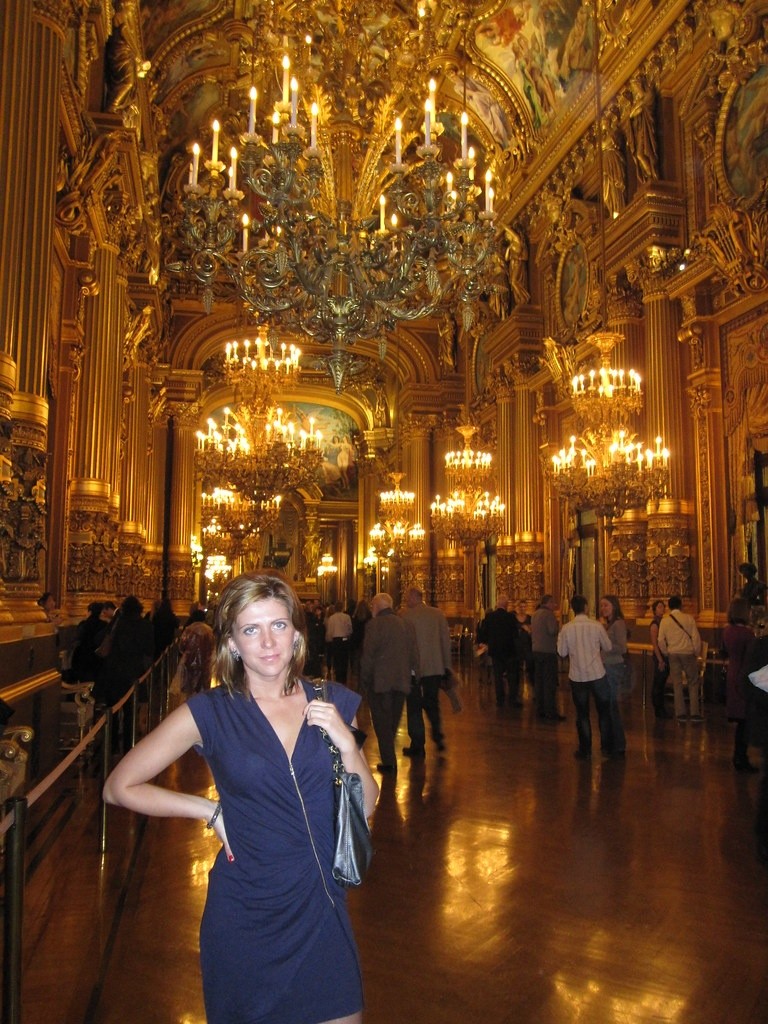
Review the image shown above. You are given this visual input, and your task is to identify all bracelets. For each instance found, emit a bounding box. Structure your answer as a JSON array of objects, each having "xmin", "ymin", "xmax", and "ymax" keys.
[{"xmin": 207, "ymin": 800, "xmax": 221, "ymax": 831}]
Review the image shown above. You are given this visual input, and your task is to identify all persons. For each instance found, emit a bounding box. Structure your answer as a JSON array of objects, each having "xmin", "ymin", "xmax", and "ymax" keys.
[
  {"xmin": 477, "ymin": 592, "xmax": 631, "ymax": 758},
  {"xmin": 435, "ymin": 73, "xmax": 663, "ymax": 366},
  {"xmin": 106, "ymin": 0, "xmax": 139, "ymax": 115},
  {"xmin": 740, "ymin": 564, "xmax": 768, "ymax": 605},
  {"xmin": 651, "ymin": 597, "xmax": 768, "ymax": 864},
  {"xmin": 37, "ymin": 587, "xmax": 452, "ymax": 773},
  {"xmin": 103, "ymin": 569, "xmax": 380, "ymax": 1024}
]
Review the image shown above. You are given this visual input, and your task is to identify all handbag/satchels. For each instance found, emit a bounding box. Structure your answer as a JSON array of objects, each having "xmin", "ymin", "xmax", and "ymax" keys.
[
  {"xmin": 313, "ymin": 678, "xmax": 373, "ymax": 887},
  {"xmin": 94, "ymin": 617, "xmax": 119, "ymax": 660}
]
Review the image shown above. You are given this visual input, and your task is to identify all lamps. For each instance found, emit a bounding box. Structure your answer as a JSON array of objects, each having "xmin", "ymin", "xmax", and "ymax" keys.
[
  {"xmin": 159, "ymin": 0, "xmax": 516, "ymax": 605},
  {"xmin": 546, "ymin": 1, "xmax": 672, "ymax": 516}
]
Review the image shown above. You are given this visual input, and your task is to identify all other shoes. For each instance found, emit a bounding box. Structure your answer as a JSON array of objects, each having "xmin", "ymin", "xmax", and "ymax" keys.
[
  {"xmin": 548, "ymin": 713, "xmax": 567, "ymax": 721},
  {"xmin": 733, "ymin": 756, "xmax": 760, "ymax": 774},
  {"xmin": 675, "ymin": 715, "xmax": 687, "ymax": 722},
  {"xmin": 509, "ymin": 702, "xmax": 523, "ymax": 708},
  {"xmin": 601, "ymin": 750, "xmax": 626, "ymax": 760},
  {"xmin": 437, "ymin": 742, "xmax": 445, "ymax": 751},
  {"xmin": 377, "ymin": 763, "xmax": 397, "ymax": 774},
  {"xmin": 574, "ymin": 750, "xmax": 592, "ymax": 758},
  {"xmin": 403, "ymin": 747, "xmax": 425, "ymax": 757},
  {"xmin": 691, "ymin": 715, "xmax": 705, "ymax": 722}
]
